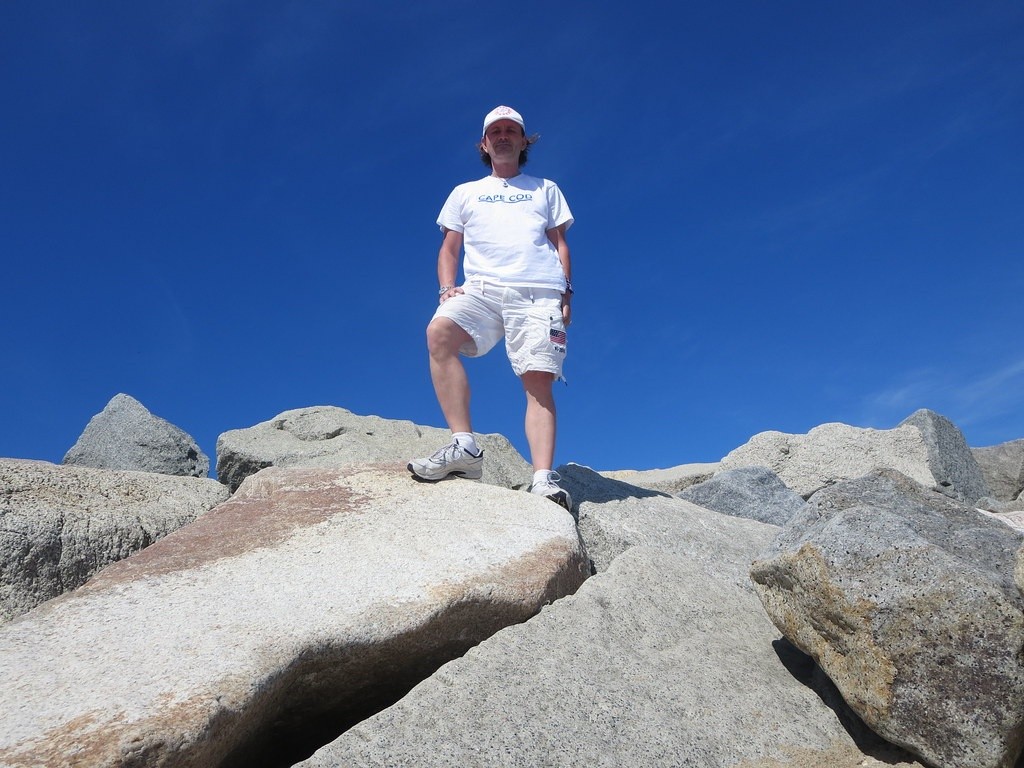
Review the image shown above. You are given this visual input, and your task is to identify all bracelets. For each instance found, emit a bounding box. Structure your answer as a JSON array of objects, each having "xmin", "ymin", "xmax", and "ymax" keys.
[{"xmin": 566, "ymin": 279, "xmax": 574, "ymax": 295}]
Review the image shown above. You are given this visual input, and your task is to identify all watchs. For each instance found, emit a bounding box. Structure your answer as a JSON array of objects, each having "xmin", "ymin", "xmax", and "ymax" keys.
[{"xmin": 437, "ymin": 286, "xmax": 455, "ymax": 296}]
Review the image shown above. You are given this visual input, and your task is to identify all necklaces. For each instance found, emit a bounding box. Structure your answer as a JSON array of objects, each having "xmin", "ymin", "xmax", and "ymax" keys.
[{"xmin": 491, "ymin": 171, "xmax": 521, "ymax": 187}]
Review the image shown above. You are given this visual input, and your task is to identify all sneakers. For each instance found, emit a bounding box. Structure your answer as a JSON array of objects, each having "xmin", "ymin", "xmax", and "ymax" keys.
[
  {"xmin": 530, "ymin": 470, "xmax": 572, "ymax": 512},
  {"xmin": 407, "ymin": 438, "xmax": 485, "ymax": 480}
]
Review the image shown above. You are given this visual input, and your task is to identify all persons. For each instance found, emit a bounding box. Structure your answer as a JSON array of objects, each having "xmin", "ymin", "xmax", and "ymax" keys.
[{"xmin": 405, "ymin": 104, "xmax": 575, "ymax": 514}]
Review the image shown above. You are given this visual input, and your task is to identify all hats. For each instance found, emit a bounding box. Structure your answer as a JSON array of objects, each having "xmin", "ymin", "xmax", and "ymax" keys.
[{"xmin": 483, "ymin": 105, "xmax": 525, "ymax": 137}]
